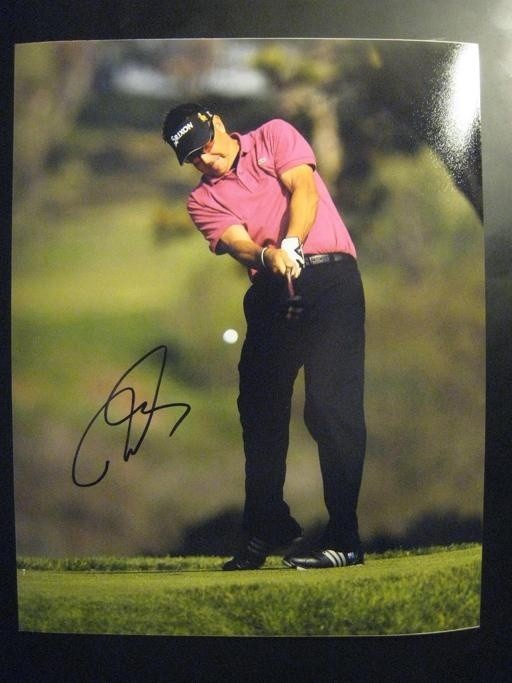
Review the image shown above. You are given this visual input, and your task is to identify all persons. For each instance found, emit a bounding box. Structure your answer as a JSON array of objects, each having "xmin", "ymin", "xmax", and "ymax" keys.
[{"xmin": 160, "ymin": 100, "xmax": 368, "ymax": 570}]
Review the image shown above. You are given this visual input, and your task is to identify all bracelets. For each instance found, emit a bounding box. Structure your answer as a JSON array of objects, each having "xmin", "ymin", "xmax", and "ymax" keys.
[{"xmin": 259, "ymin": 245, "xmax": 269, "ymax": 271}]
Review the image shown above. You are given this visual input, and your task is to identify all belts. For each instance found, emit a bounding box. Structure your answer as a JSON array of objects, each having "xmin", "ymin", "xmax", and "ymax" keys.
[{"xmin": 304, "ymin": 251, "xmax": 345, "ymax": 267}]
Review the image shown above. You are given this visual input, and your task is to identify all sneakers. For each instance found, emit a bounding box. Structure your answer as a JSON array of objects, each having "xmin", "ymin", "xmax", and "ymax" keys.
[
  {"xmin": 222, "ymin": 516, "xmax": 304, "ymax": 570},
  {"xmin": 281, "ymin": 544, "xmax": 365, "ymax": 568}
]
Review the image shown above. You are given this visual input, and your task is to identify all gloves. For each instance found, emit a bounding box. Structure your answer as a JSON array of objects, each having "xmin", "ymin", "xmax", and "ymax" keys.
[{"xmin": 278, "ymin": 235, "xmax": 309, "ymax": 280}]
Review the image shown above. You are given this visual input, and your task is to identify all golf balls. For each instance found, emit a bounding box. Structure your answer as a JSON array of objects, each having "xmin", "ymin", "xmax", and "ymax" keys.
[{"xmin": 223, "ymin": 329, "xmax": 238, "ymax": 344}]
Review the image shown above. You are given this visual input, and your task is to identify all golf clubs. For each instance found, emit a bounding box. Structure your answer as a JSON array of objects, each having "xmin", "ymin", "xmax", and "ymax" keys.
[{"xmin": 285, "ymin": 268, "xmax": 303, "ymax": 318}]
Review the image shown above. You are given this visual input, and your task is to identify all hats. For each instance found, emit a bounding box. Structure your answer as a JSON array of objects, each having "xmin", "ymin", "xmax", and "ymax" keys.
[{"xmin": 159, "ymin": 103, "xmax": 213, "ymax": 166}]
